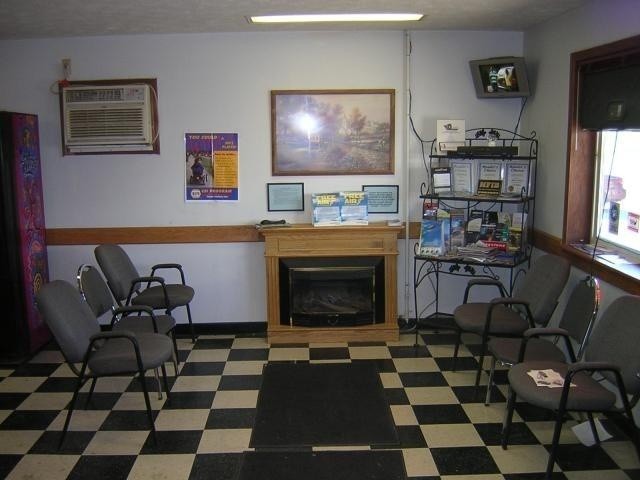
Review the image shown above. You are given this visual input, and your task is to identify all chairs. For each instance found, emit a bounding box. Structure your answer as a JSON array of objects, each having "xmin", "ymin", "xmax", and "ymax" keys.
[
  {"xmin": 94, "ymin": 243, "xmax": 197, "ymax": 364},
  {"xmin": 501, "ymin": 295, "xmax": 640, "ymax": 479},
  {"xmin": 36, "ymin": 279, "xmax": 175, "ymax": 452},
  {"xmin": 485, "ymin": 277, "xmax": 600, "ymax": 436},
  {"xmin": 78, "ymin": 264, "xmax": 179, "ymax": 400},
  {"xmin": 455, "ymin": 254, "xmax": 571, "ymax": 390}
]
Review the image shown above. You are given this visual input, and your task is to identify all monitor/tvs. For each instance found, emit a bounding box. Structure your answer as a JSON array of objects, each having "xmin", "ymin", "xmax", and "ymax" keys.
[{"xmin": 467, "ymin": 55, "xmax": 535, "ymax": 99}]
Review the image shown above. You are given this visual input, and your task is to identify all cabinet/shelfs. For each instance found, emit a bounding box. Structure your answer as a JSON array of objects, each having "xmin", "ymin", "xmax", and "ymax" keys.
[{"xmin": 413, "ymin": 128, "xmax": 538, "ymax": 346}]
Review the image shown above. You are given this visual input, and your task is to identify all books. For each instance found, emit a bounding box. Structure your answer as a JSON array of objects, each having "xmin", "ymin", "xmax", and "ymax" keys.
[
  {"xmin": 338, "ymin": 190, "xmax": 369, "ymax": 225},
  {"xmin": 417, "ymin": 158, "xmax": 533, "ymax": 266},
  {"xmin": 436, "ymin": 119, "xmax": 466, "ymax": 156},
  {"xmin": 309, "ymin": 191, "xmax": 342, "ymax": 227}
]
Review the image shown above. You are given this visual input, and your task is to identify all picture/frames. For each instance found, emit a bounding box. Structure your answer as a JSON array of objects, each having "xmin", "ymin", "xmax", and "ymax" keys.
[
  {"xmin": 362, "ymin": 185, "xmax": 398, "ymax": 214},
  {"xmin": 270, "ymin": 89, "xmax": 395, "ymax": 174},
  {"xmin": 267, "ymin": 182, "xmax": 304, "ymax": 212}
]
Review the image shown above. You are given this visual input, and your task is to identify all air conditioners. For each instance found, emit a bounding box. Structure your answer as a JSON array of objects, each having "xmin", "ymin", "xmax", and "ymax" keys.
[{"xmin": 61, "ymin": 84, "xmax": 153, "ymax": 152}]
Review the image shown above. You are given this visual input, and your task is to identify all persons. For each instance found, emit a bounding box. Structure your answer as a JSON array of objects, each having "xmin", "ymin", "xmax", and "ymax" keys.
[{"xmin": 191, "ymin": 157, "xmax": 205, "ymax": 184}]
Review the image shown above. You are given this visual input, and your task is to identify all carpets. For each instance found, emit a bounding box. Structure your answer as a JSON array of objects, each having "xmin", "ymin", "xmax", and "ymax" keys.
[
  {"xmin": 232, "ymin": 450, "xmax": 409, "ymax": 478},
  {"xmin": 249, "ymin": 361, "xmax": 400, "ymax": 448}
]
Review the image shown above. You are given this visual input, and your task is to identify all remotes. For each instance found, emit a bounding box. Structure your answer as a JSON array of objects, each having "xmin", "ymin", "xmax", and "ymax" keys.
[{"xmin": 260, "ymin": 220, "xmax": 286, "ymax": 225}]
